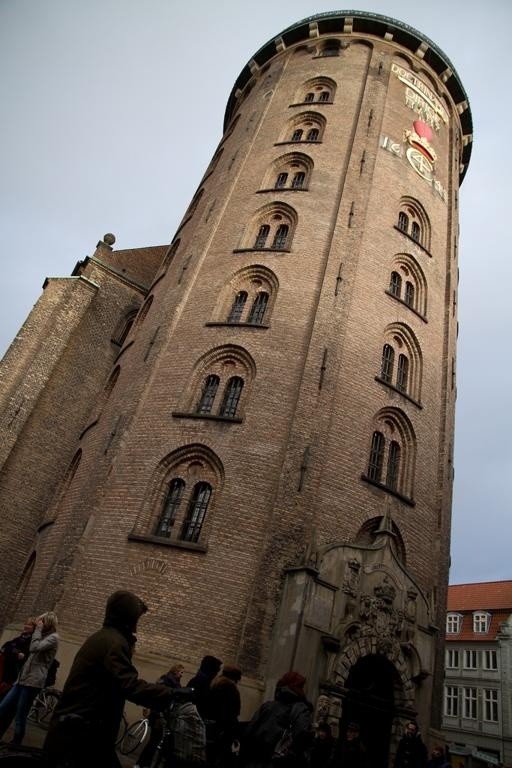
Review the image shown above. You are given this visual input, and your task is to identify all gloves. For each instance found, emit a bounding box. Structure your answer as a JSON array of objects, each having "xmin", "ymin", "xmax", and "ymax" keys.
[{"xmin": 176, "ymin": 688, "xmax": 195, "ymax": 704}]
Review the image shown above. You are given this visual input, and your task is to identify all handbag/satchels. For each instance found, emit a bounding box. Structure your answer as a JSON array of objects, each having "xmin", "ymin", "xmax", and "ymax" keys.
[
  {"xmin": 273, "ymin": 729, "xmax": 310, "ymax": 766},
  {"xmin": 166, "ymin": 703, "xmax": 207, "ymax": 761}
]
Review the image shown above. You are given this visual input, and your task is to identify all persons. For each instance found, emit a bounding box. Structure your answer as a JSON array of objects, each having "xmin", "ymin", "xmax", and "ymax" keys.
[
  {"xmin": 0, "ymin": 612, "xmax": 59, "ymax": 753},
  {"xmin": 138, "ymin": 664, "xmax": 184, "ymax": 768},
  {"xmin": 309, "ymin": 722, "xmax": 336, "ymax": 768},
  {"xmin": 239, "ymin": 671, "xmax": 317, "ymax": 768},
  {"xmin": 393, "ymin": 722, "xmax": 428, "ymax": 768},
  {"xmin": 42, "ymin": 590, "xmax": 192, "ymax": 768},
  {"xmin": 333, "ymin": 723, "xmax": 368, "ymax": 768},
  {"xmin": 429, "ymin": 745, "xmax": 452, "ymax": 768},
  {"xmin": 181, "ymin": 656, "xmax": 241, "ymax": 768},
  {"xmin": 0, "ymin": 616, "xmax": 37, "ymax": 704}
]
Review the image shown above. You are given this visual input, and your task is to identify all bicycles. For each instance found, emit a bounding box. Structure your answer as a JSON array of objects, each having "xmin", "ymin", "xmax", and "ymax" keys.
[
  {"xmin": 35, "ymin": 687, "xmax": 129, "ymax": 747},
  {"xmin": 27, "ymin": 686, "xmax": 54, "ymax": 723},
  {"xmin": 120, "ymin": 708, "xmax": 152, "ymax": 756}
]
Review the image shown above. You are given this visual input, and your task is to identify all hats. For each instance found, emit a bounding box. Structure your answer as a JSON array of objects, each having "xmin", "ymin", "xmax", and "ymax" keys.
[{"xmin": 277, "ymin": 672, "xmax": 305, "ymax": 696}]
